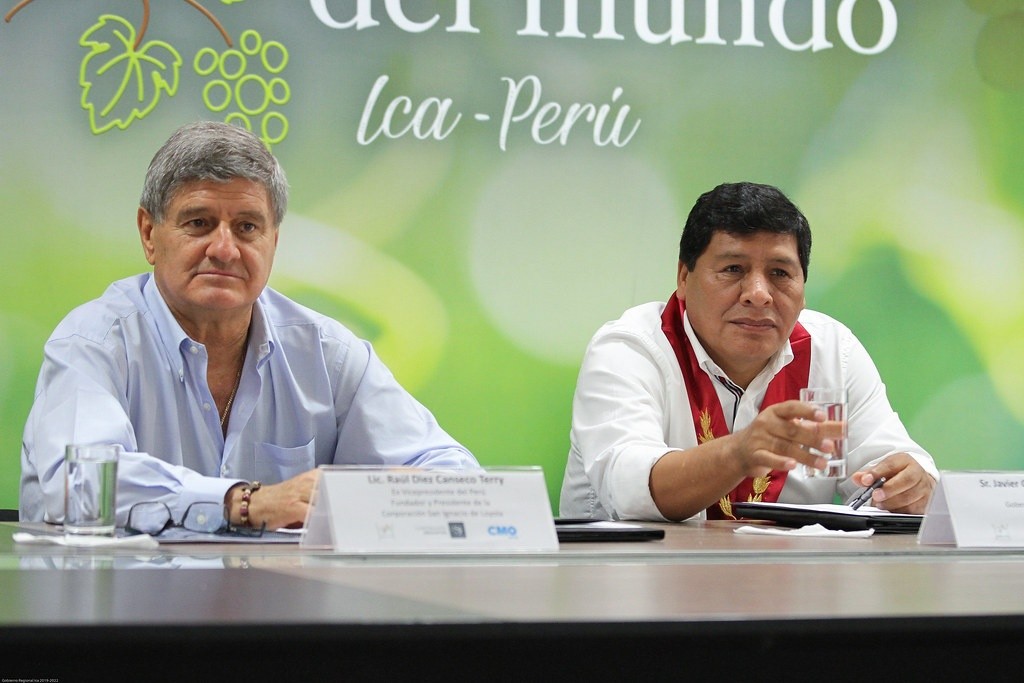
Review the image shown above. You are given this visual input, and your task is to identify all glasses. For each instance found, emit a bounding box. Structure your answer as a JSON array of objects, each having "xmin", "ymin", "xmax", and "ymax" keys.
[{"xmin": 124, "ymin": 500, "xmax": 266, "ymax": 537}]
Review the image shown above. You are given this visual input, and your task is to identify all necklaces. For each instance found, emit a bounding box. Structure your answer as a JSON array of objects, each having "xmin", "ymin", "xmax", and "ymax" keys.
[{"xmin": 218, "ymin": 344, "xmax": 246, "ymax": 425}]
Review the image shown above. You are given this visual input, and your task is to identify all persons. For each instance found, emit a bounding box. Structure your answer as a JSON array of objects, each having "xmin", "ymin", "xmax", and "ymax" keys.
[
  {"xmin": 19, "ymin": 121, "xmax": 486, "ymax": 529},
  {"xmin": 554, "ymin": 179, "xmax": 940, "ymax": 527}
]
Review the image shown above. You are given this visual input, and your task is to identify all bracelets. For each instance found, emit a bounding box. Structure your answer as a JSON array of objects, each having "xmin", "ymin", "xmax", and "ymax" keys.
[{"xmin": 239, "ymin": 480, "xmax": 263, "ymax": 530}]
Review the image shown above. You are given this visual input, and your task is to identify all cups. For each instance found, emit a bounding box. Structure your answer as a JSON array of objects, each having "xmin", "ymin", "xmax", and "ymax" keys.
[
  {"xmin": 800, "ymin": 387, "xmax": 847, "ymax": 478},
  {"xmin": 63, "ymin": 444, "xmax": 120, "ymax": 536}
]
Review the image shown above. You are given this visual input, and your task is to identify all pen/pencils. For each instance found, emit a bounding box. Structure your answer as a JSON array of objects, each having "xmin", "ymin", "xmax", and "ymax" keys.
[{"xmin": 851, "ymin": 476, "xmax": 886, "ymax": 511}]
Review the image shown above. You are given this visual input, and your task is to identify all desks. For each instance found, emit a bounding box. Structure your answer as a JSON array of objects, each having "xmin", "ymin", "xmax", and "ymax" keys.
[{"xmin": 0, "ymin": 520, "xmax": 1024, "ymax": 683}]
